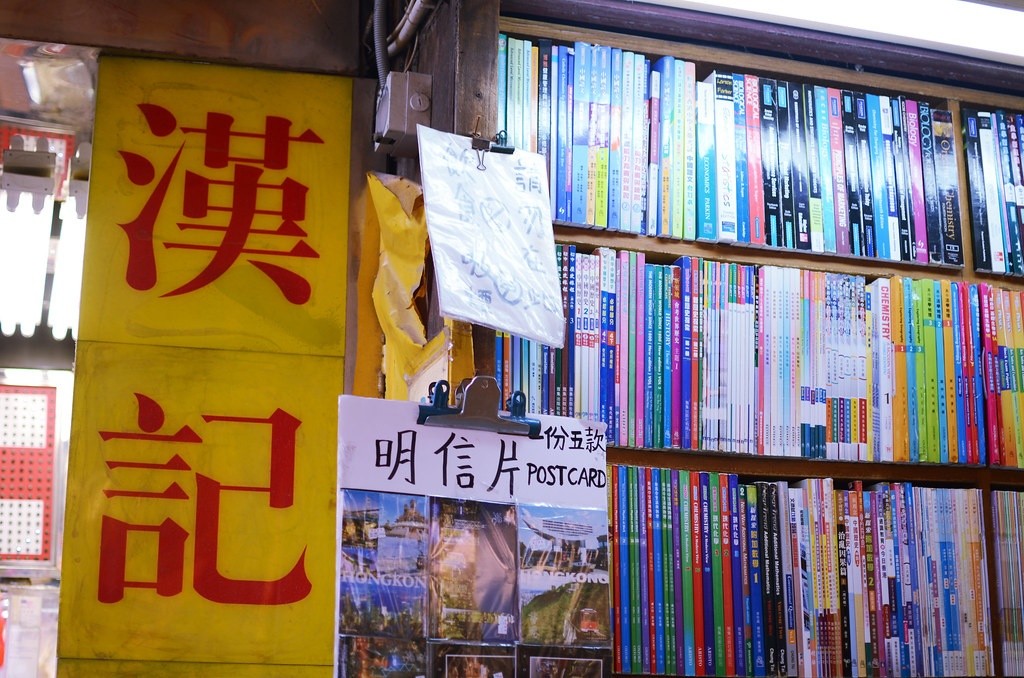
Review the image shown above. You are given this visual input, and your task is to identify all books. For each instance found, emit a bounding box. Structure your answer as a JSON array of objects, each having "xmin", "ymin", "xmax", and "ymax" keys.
[
  {"xmin": 494, "ymin": 245, "xmax": 1024, "ymax": 470},
  {"xmin": 498, "ymin": 33, "xmax": 1024, "ymax": 277},
  {"xmin": 607, "ymin": 465, "xmax": 1024, "ymax": 678}
]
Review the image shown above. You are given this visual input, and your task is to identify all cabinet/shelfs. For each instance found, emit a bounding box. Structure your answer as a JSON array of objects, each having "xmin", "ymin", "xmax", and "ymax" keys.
[{"xmin": 461, "ymin": 15, "xmax": 1024, "ymax": 678}]
